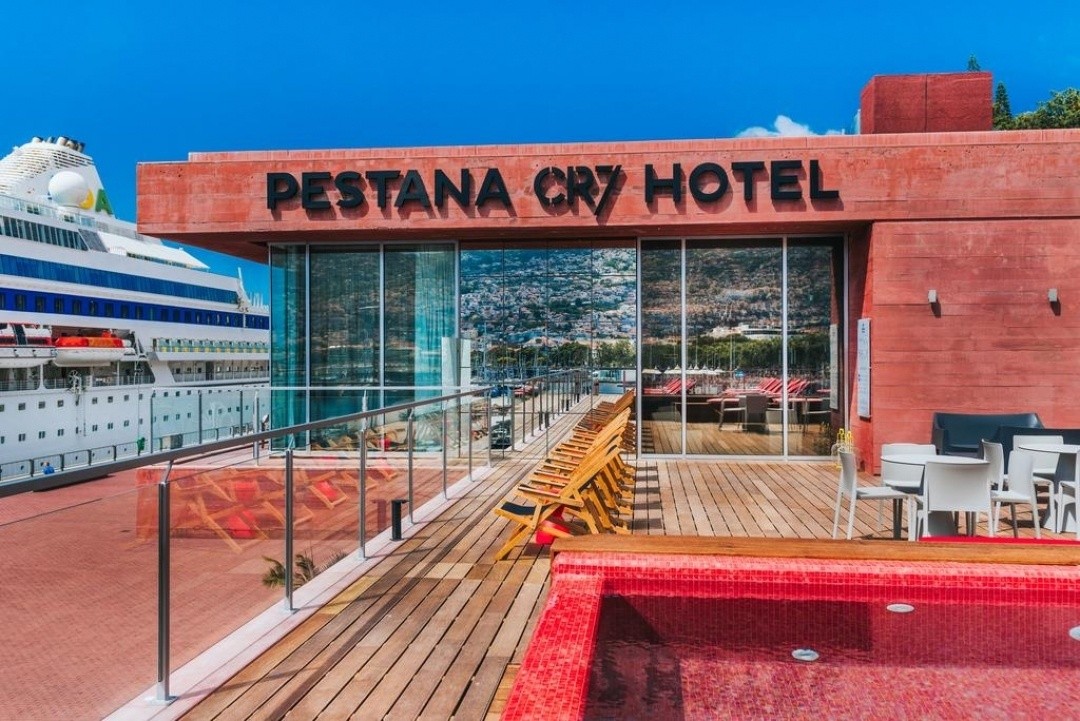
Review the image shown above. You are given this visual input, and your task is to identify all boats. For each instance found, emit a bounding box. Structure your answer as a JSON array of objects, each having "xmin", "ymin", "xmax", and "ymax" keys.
[
  {"xmin": 0, "ymin": 324, "xmax": 60, "ymax": 369},
  {"xmin": 53, "ymin": 331, "xmax": 148, "ymax": 369}
]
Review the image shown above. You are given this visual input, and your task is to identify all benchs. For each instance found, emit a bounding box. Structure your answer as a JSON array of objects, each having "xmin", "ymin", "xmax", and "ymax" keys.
[
  {"xmin": 992, "ymin": 424, "xmax": 1080, "ymax": 515},
  {"xmin": 930, "ymin": 411, "xmax": 1047, "ymax": 459}
]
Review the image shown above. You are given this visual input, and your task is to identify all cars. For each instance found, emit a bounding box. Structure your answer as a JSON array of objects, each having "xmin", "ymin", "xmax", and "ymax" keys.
[{"xmin": 480, "ymin": 382, "xmax": 538, "ymax": 400}]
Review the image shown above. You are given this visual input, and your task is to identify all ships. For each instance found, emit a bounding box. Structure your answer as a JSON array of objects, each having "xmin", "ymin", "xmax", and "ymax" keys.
[{"xmin": 0, "ymin": 134, "xmax": 297, "ymax": 498}]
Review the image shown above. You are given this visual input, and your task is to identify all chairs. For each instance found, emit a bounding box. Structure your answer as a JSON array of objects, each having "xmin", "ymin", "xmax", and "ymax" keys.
[
  {"xmin": 912, "ymin": 459, "xmax": 995, "ymax": 542},
  {"xmin": 981, "ymin": 438, "xmax": 1058, "ymax": 533},
  {"xmin": 133, "ymin": 424, "xmax": 407, "ymax": 556},
  {"xmin": 878, "ymin": 443, "xmax": 938, "ymax": 532},
  {"xmin": 572, "ymin": 387, "xmax": 638, "ymax": 453},
  {"xmin": 830, "ymin": 445, "xmax": 913, "ymax": 542},
  {"xmin": 1056, "ymin": 448, "xmax": 1080, "ymax": 541},
  {"xmin": 1013, "ymin": 434, "xmax": 1064, "ymax": 506},
  {"xmin": 490, "ymin": 407, "xmax": 639, "ymax": 563},
  {"xmin": 971, "ymin": 451, "xmax": 1043, "ymax": 538},
  {"xmin": 643, "ymin": 375, "xmax": 810, "ymax": 431}
]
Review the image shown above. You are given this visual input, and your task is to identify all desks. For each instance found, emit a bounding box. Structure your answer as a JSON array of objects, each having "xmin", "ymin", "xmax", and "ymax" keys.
[
  {"xmin": 772, "ymin": 396, "xmax": 825, "ymax": 434},
  {"xmin": 1017, "ymin": 442, "xmax": 1080, "ymax": 533},
  {"xmin": 882, "ymin": 453, "xmax": 991, "ymax": 542},
  {"xmin": 817, "ymin": 387, "xmax": 831, "ymax": 430}
]
